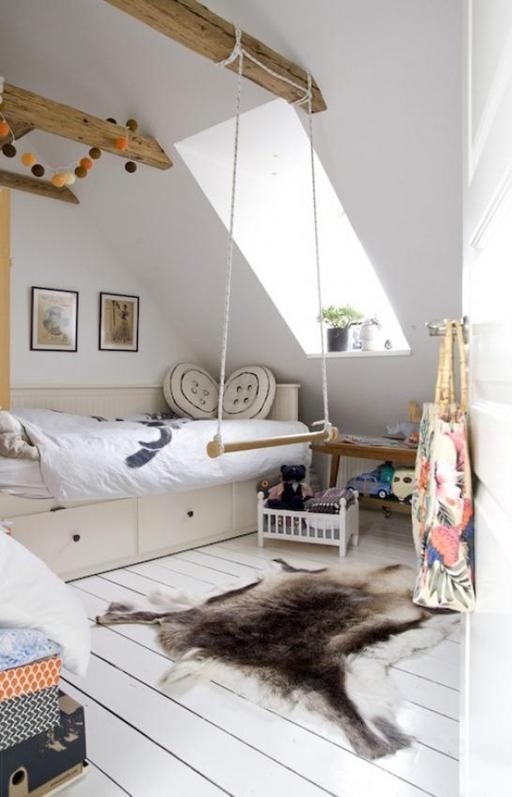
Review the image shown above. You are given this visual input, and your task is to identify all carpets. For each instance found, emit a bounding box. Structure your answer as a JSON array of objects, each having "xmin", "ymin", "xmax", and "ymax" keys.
[{"xmin": 95, "ymin": 555, "xmax": 460, "ymax": 762}]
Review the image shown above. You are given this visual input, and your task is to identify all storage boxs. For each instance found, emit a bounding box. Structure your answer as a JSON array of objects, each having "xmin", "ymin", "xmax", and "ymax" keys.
[{"xmin": 0, "ymin": 655, "xmax": 87, "ymax": 795}]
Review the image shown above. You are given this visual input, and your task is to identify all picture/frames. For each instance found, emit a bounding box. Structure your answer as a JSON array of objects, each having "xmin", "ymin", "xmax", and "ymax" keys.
[{"xmin": 30, "ymin": 285, "xmax": 139, "ymax": 352}]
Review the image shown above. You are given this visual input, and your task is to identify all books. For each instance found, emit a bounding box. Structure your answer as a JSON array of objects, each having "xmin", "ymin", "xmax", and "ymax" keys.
[
  {"xmin": 344, "ymin": 433, "xmax": 398, "ymax": 443},
  {"xmin": 345, "ymin": 438, "xmax": 397, "ymax": 447}
]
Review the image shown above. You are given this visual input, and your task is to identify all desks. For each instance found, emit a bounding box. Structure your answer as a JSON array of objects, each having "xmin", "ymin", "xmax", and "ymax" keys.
[{"xmin": 309, "ymin": 439, "xmax": 417, "ymax": 516}]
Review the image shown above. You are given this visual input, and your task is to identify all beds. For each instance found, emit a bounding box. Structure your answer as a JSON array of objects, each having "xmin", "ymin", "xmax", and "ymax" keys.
[{"xmin": 1, "ymin": 419, "xmax": 313, "ymax": 584}]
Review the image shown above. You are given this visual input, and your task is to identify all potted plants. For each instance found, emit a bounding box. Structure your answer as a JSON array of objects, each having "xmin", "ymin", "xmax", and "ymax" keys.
[{"xmin": 318, "ymin": 303, "xmax": 364, "ymax": 352}]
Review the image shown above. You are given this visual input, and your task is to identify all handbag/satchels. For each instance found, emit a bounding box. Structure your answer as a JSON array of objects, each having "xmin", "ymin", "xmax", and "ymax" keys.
[{"xmin": 411, "ymin": 400, "xmax": 475, "ymax": 612}]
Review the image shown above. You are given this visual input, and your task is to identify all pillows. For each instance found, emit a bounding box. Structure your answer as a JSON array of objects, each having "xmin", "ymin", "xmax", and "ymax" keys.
[
  {"xmin": 1, "ymin": 531, "xmax": 92, "ymax": 678},
  {"xmin": 162, "ymin": 359, "xmax": 276, "ymax": 418}
]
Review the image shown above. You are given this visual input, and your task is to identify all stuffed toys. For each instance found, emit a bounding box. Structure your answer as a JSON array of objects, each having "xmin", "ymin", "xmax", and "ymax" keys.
[{"xmin": 267, "ymin": 464, "xmax": 314, "ymax": 508}]
